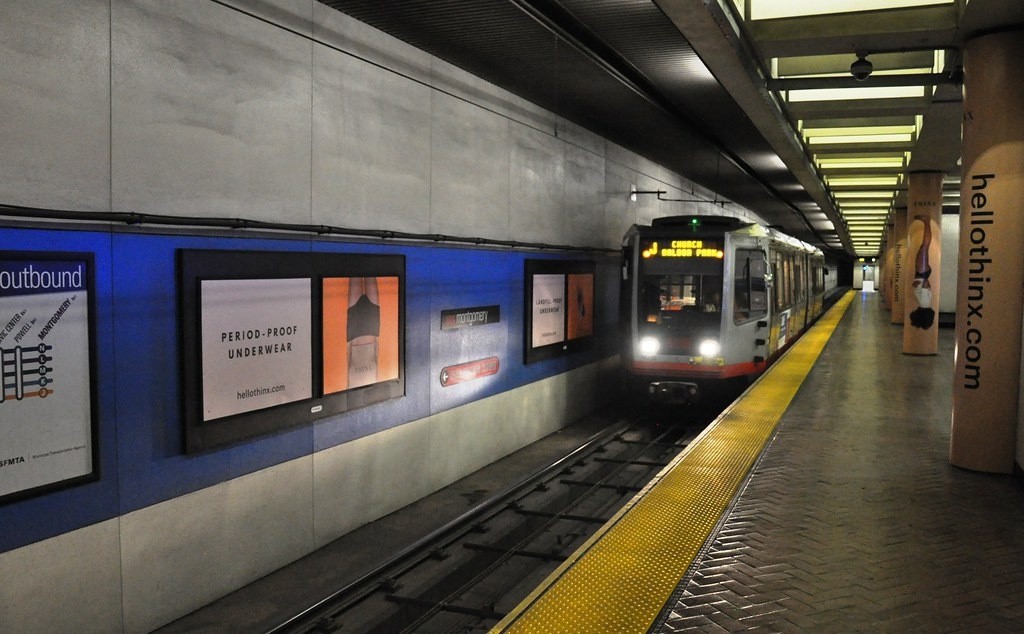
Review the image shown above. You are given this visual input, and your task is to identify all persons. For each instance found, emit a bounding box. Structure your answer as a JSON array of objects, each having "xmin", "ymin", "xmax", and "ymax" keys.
[
  {"xmin": 346, "ymin": 277, "xmax": 380, "ymax": 389},
  {"xmin": 909, "ymin": 215, "xmax": 936, "ymax": 329},
  {"xmin": 710, "ymin": 289, "xmax": 747, "ymax": 322}
]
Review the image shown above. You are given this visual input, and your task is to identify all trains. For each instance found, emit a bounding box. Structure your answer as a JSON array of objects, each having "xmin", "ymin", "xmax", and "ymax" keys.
[{"xmin": 620, "ymin": 214, "xmax": 830, "ymax": 412}]
